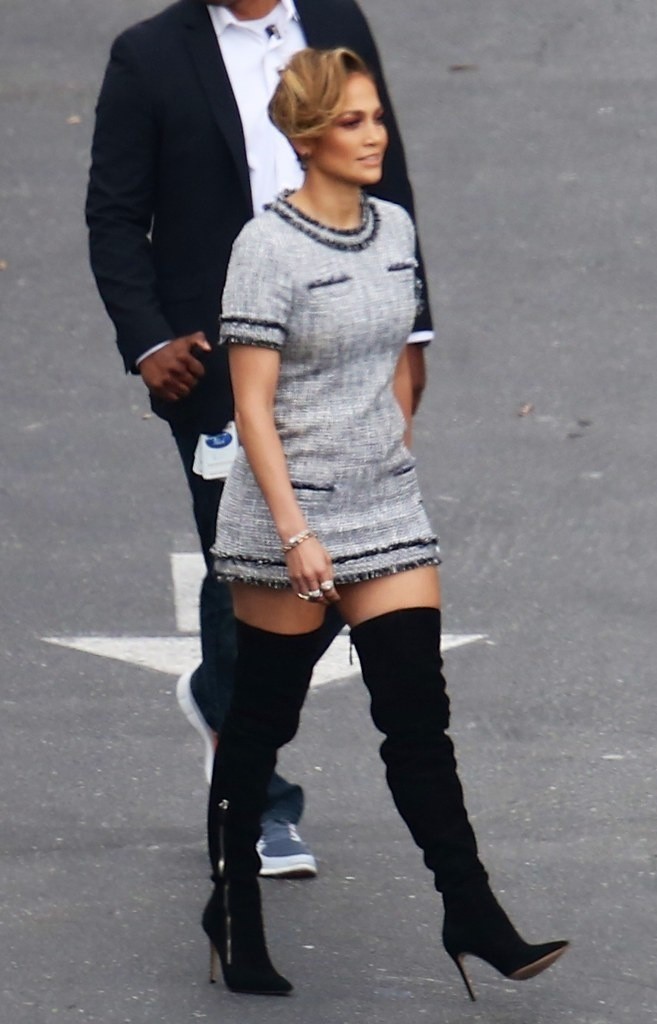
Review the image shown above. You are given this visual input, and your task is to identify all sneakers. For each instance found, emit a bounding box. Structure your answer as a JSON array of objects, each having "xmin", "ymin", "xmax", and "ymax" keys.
[
  {"xmin": 177, "ymin": 661, "xmax": 217, "ymax": 785},
  {"xmin": 255, "ymin": 818, "xmax": 318, "ymax": 876}
]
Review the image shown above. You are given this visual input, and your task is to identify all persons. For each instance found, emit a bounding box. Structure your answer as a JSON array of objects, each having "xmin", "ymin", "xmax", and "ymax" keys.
[
  {"xmin": 84, "ymin": 0, "xmax": 434, "ymax": 879},
  {"xmin": 200, "ymin": 47, "xmax": 570, "ymax": 1002}
]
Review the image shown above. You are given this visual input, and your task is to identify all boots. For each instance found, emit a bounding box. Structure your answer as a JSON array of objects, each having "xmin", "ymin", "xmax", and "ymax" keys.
[
  {"xmin": 349, "ymin": 607, "xmax": 570, "ymax": 1000},
  {"xmin": 201, "ymin": 612, "xmax": 323, "ymax": 995}
]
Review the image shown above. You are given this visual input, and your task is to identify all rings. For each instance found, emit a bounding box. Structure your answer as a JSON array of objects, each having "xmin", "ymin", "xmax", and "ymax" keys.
[{"xmin": 296, "ymin": 579, "xmax": 334, "ymax": 602}]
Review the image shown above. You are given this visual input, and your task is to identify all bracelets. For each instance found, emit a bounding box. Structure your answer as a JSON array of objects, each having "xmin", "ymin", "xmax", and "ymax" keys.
[{"xmin": 281, "ymin": 529, "xmax": 315, "ymax": 554}]
